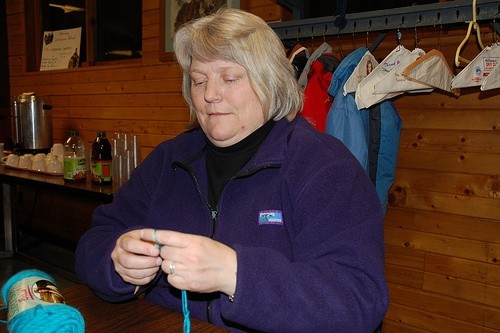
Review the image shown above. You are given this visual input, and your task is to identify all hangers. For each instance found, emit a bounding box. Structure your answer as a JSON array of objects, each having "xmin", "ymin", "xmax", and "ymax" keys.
[
  {"xmin": 403, "ymin": 20, "xmax": 461, "ymax": 96},
  {"xmin": 455, "ymin": 0, "xmax": 485, "ymax": 67}
]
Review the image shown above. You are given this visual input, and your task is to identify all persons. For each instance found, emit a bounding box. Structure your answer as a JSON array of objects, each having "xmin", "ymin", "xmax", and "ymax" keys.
[{"xmin": 76, "ymin": 7, "xmax": 390, "ymax": 333}]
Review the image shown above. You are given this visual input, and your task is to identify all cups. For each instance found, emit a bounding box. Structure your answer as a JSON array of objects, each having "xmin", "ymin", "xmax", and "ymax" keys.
[
  {"xmin": 110, "ymin": 132, "xmax": 140, "ymax": 183},
  {"xmin": 1, "ymin": 143, "xmax": 64, "ymax": 175}
]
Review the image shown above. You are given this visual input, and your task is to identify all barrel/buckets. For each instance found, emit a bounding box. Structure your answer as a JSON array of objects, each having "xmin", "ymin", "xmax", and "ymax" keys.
[{"xmin": 11, "ymin": 92, "xmax": 53, "ymax": 153}]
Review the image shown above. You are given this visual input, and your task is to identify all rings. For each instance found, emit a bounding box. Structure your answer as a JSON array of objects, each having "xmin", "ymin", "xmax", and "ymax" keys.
[{"xmin": 168, "ymin": 262, "xmax": 176, "ymax": 276}]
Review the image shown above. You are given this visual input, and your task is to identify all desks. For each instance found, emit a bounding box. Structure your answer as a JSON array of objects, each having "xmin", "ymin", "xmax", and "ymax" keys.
[
  {"xmin": 0, "ymin": 164, "xmax": 113, "ymax": 255},
  {"xmin": 0, "ymin": 282, "xmax": 236, "ymax": 333}
]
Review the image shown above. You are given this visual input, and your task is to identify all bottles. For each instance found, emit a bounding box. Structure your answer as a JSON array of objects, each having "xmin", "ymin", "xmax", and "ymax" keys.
[
  {"xmin": 91, "ymin": 131, "xmax": 115, "ymax": 187},
  {"xmin": 63, "ymin": 130, "xmax": 88, "ymax": 184}
]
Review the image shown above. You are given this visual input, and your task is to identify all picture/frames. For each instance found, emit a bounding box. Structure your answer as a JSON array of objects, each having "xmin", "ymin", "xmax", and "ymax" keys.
[{"xmin": 158, "ymin": 0, "xmax": 249, "ymax": 62}]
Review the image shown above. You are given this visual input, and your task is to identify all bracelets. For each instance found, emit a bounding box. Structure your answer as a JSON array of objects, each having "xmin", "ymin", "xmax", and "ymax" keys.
[{"xmin": 229, "ymin": 293, "xmax": 236, "ymax": 303}]
